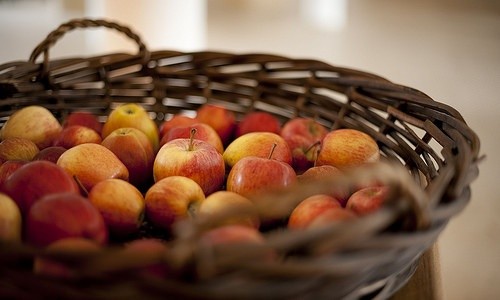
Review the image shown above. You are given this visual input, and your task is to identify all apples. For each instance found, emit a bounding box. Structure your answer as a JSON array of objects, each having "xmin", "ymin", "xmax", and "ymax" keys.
[{"xmin": 0, "ymin": 105, "xmax": 390, "ymax": 249}]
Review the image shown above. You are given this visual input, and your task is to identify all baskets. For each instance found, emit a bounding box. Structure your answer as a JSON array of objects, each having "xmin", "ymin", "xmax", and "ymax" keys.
[{"xmin": 0, "ymin": 16, "xmax": 487, "ymax": 300}]
